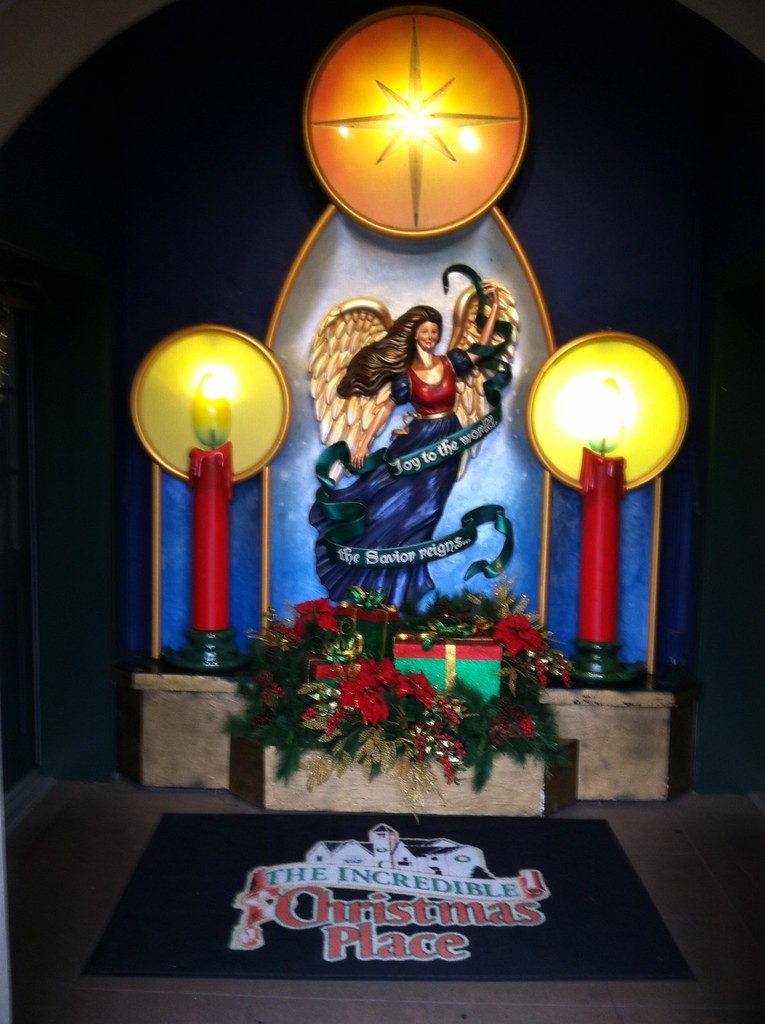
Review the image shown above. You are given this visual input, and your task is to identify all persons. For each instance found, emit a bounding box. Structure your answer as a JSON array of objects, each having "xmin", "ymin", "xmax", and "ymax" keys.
[{"xmin": 310, "ymin": 281, "xmax": 499, "ymax": 622}]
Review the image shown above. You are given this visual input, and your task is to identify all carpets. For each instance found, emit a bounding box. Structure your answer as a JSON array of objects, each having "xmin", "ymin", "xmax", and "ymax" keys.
[{"xmin": 70, "ymin": 808, "xmax": 701, "ymax": 988}]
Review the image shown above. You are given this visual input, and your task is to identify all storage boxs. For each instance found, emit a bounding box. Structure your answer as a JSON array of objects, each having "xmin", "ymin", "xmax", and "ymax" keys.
[
  {"xmin": 392, "ymin": 628, "xmax": 502, "ymax": 711},
  {"xmin": 305, "ymin": 646, "xmax": 377, "ymax": 679},
  {"xmin": 333, "ymin": 599, "xmax": 397, "ymax": 659}
]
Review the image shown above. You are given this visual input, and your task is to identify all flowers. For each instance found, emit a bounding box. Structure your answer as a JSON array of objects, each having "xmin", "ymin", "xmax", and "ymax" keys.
[{"xmin": 232, "ymin": 554, "xmax": 578, "ymax": 818}]
